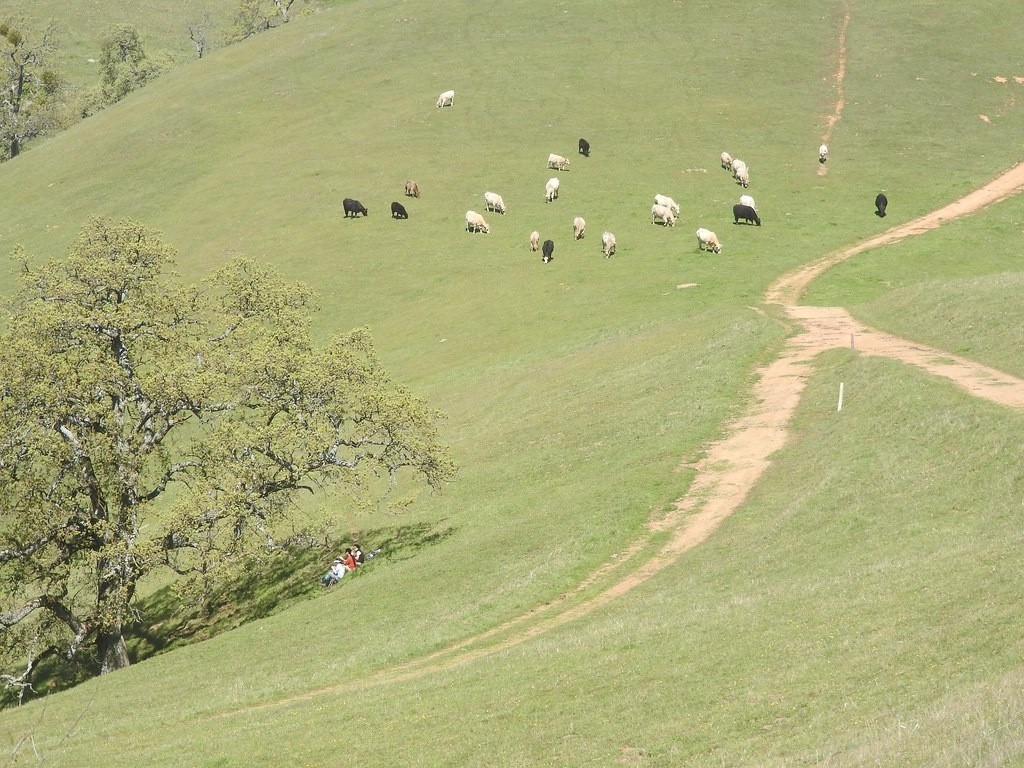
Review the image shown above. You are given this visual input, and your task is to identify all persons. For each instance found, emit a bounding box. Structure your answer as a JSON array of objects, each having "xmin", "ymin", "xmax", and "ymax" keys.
[
  {"xmin": 320, "ymin": 558, "xmax": 346, "ymax": 589},
  {"xmin": 342, "ymin": 548, "xmax": 357, "ymax": 572},
  {"xmin": 352, "ymin": 544, "xmax": 364, "ymax": 565}
]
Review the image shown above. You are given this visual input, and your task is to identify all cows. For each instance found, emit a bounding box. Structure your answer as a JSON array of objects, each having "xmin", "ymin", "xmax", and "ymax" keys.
[
  {"xmin": 573, "ymin": 217, "xmax": 586, "ymax": 240},
  {"xmin": 530, "ymin": 231, "xmax": 539, "ymax": 253},
  {"xmin": 818, "ymin": 144, "xmax": 829, "ymax": 163},
  {"xmin": 720, "ymin": 152, "xmax": 750, "ymax": 188},
  {"xmin": 543, "ymin": 177, "xmax": 559, "ymax": 203},
  {"xmin": 466, "ymin": 211, "xmax": 489, "ymax": 234},
  {"xmin": 654, "ymin": 194, "xmax": 680, "ymax": 215},
  {"xmin": 542, "ymin": 240, "xmax": 554, "ymax": 264},
  {"xmin": 875, "ymin": 193, "xmax": 888, "ymax": 218},
  {"xmin": 391, "ymin": 202, "xmax": 408, "ymax": 219},
  {"xmin": 696, "ymin": 228, "xmax": 723, "ymax": 254},
  {"xmin": 484, "ymin": 192, "xmax": 507, "ymax": 215},
  {"xmin": 602, "ymin": 232, "xmax": 616, "ymax": 258},
  {"xmin": 733, "ymin": 195, "xmax": 761, "ymax": 226},
  {"xmin": 547, "ymin": 153, "xmax": 569, "ymax": 171},
  {"xmin": 404, "ymin": 180, "xmax": 420, "ymax": 199},
  {"xmin": 651, "ymin": 205, "xmax": 675, "ymax": 227},
  {"xmin": 579, "ymin": 138, "xmax": 590, "ymax": 157},
  {"xmin": 436, "ymin": 90, "xmax": 454, "ymax": 108},
  {"xmin": 343, "ymin": 198, "xmax": 367, "ymax": 219}
]
